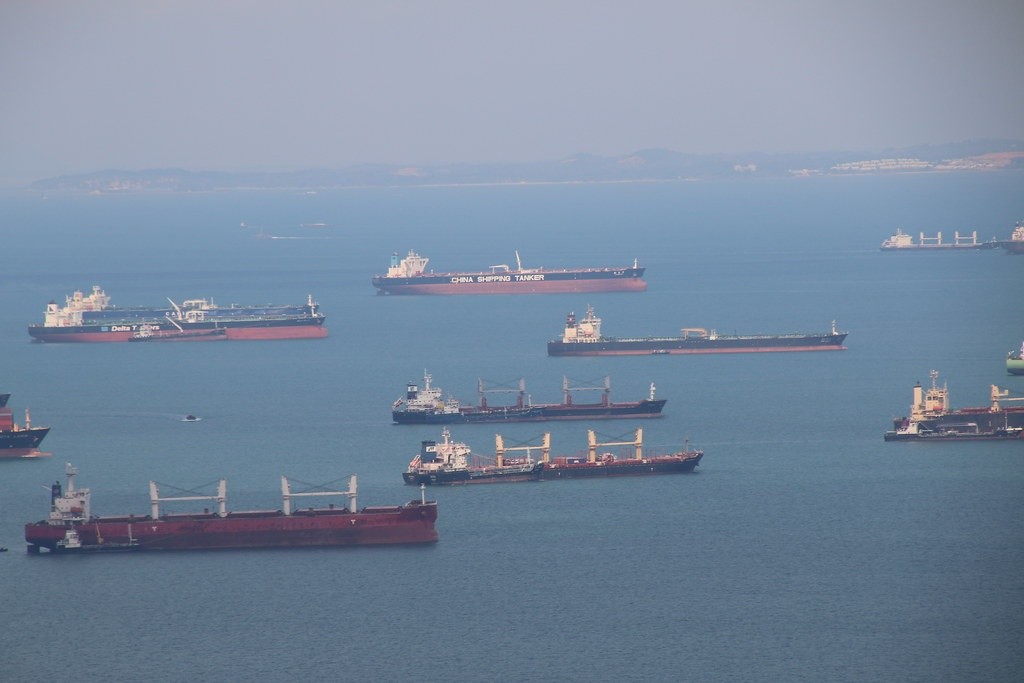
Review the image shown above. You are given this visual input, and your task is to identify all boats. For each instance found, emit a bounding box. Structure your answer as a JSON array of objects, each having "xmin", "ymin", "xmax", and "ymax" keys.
[
  {"xmin": 24, "ymin": 463, "xmax": 439, "ymax": 555},
  {"xmin": 547, "ymin": 301, "xmax": 849, "ymax": 356},
  {"xmin": 999, "ymin": 222, "xmax": 1023, "ymax": 256},
  {"xmin": 393, "ymin": 368, "xmax": 668, "ymax": 426},
  {"xmin": 371, "ymin": 248, "xmax": 647, "ymax": 295},
  {"xmin": 1006, "ymin": 339, "xmax": 1024, "ymax": 374},
  {"xmin": 0, "ymin": 393, "xmax": 50, "ymax": 458},
  {"xmin": 27, "ymin": 284, "xmax": 327, "ymax": 341},
  {"xmin": 402, "ymin": 426, "xmax": 705, "ymax": 487},
  {"xmin": 883, "ymin": 369, "xmax": 1024, "ymax": 441},
  {"xmin": 879, "ymin": 230, "xmax": 998, "ymax": 250}
]
